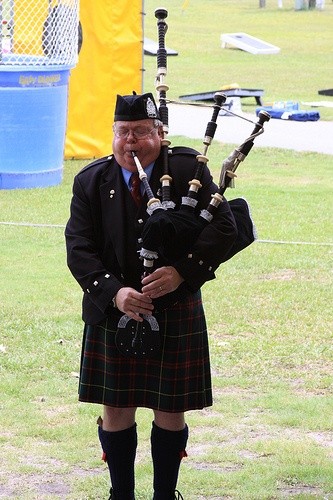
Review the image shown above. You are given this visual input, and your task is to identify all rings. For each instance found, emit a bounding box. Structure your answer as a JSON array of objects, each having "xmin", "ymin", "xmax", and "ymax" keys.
[{"xmin": 159, "ymin": 286, "xmax": 163, "ymax": 291}]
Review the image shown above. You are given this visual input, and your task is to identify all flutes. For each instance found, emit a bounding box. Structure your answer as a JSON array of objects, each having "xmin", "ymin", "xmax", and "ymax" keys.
[{"xmin": 115, "ymin": 8, "xmax": 271, "ymax": 356}]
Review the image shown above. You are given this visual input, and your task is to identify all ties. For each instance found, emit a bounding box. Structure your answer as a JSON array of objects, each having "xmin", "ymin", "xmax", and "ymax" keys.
[{"xmin": 130, "ymin": 173, "xmax": 143, "ymax": 210}]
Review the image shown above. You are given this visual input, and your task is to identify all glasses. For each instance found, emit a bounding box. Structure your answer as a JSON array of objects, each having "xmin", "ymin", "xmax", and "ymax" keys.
[{"xmin": 113, "ymin": 124, "xmax": 157, "ymax": 139}]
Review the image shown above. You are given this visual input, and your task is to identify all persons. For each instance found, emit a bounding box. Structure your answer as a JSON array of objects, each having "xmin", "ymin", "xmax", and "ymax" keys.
[{"xmin": 64, "ymin": 91, "xmax": 257, "ymax": 500}]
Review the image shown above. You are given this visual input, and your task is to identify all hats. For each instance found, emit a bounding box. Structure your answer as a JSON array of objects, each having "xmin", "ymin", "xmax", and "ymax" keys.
[{"xmin": 114, "ymin": 90, "xmax": 158, "ymax": 122}]
